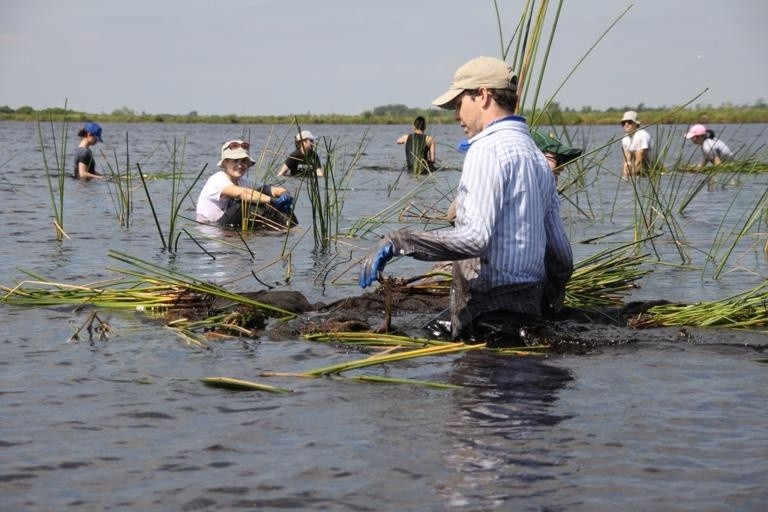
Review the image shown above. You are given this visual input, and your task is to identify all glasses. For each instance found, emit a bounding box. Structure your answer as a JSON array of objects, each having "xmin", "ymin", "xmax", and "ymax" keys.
[{"xmin": 223, "ymin": 142, "xmax": 250, "ymax": 152}]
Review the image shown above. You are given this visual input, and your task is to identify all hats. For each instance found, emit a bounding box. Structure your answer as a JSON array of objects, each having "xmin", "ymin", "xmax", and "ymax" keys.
[
  {"xmin": 84, "ymin": 123, "xmax": 102, "ymax": 142},
  {"xmin": 432, "ymin": 56, "xmax": 518, "ymax": 110},
  {"xmin": 686, "ymin": 123, "xmax": 706, "ymax": 139},
  {"xmin": 531, "ymin": 129, "xmax": 583, "ymax": 161},
  {"xmin": 296, "ymin": 130, "xmax": 318, "ymax": 142},
  {"xmin": 622, "ymin": 111, "xmax": 640, "ymax": 125},
  {"xmin": 217, "ymin": 139, "xmax": 256, "ymax": 168}
]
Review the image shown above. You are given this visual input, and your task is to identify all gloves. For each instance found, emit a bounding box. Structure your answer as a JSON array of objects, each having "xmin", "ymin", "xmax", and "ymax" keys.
[
  {"xmin": 270, "ymin": 192, "xmax": 292, "ymax": 212},
  {"xmin": 359, "ymin": 238, "xmax": 394, "ymax": 288}
]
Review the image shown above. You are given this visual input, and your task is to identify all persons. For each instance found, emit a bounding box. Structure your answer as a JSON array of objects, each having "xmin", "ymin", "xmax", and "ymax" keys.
[
  {"xmin": 683, "ymin": 123, "xmax": 736, "ymax": 169},
  {"xmin": 395, "ymin": 115, "xmax": 436, "ymax": 175},
  {"xmin": 74, "ymin": 122, "xmax": 115, "ymax": 182},
  {"xmin": 357, "ymin": 54, "xmax": 575, "ymax": 347},
  {"xmin": 195, "ymin": 138, "xmax": 299, "ymax": 226},
  {"xmin": 277, "ymin": 130, "xmax": 325, "ymax": 178},
  {"xmin": 620, "ymin": 110, "xmax": 653, "ymax": 181},
  {"xmin": 445, "ymin": 127, "xmax": 585, "ymax": 230}
]
